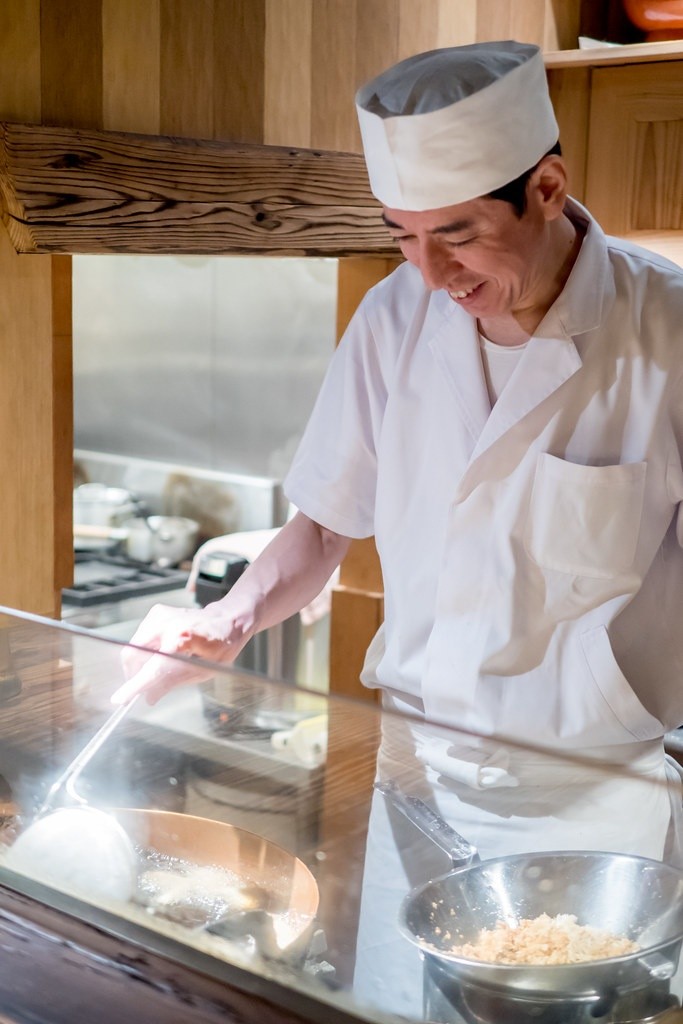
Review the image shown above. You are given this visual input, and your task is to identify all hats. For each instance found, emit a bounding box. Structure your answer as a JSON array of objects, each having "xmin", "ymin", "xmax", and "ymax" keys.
[{"xmin": 355, "ymin": 40, "xmax": 562, "ymax": 213}]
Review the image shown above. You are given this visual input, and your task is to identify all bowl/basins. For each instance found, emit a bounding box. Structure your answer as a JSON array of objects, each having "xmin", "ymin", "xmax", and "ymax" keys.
[{"xmin": 125, "ymin": 516, "xmax": 199, "ymax": 563}]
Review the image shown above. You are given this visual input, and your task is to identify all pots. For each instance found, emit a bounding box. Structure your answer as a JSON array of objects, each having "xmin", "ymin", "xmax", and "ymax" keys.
[
  {"xmin": 374, "ymin": 780, "xmax": 683, "ymax": 1007},
  {"xmin": 0, "ymin": 808, "xmax": 319, "ymax": 969}
]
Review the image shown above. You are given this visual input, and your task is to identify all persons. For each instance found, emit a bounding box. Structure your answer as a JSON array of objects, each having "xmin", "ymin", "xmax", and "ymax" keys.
[{"xmin": 118, "ymin": 39, "xmax": 683, "ymax": 870}]
